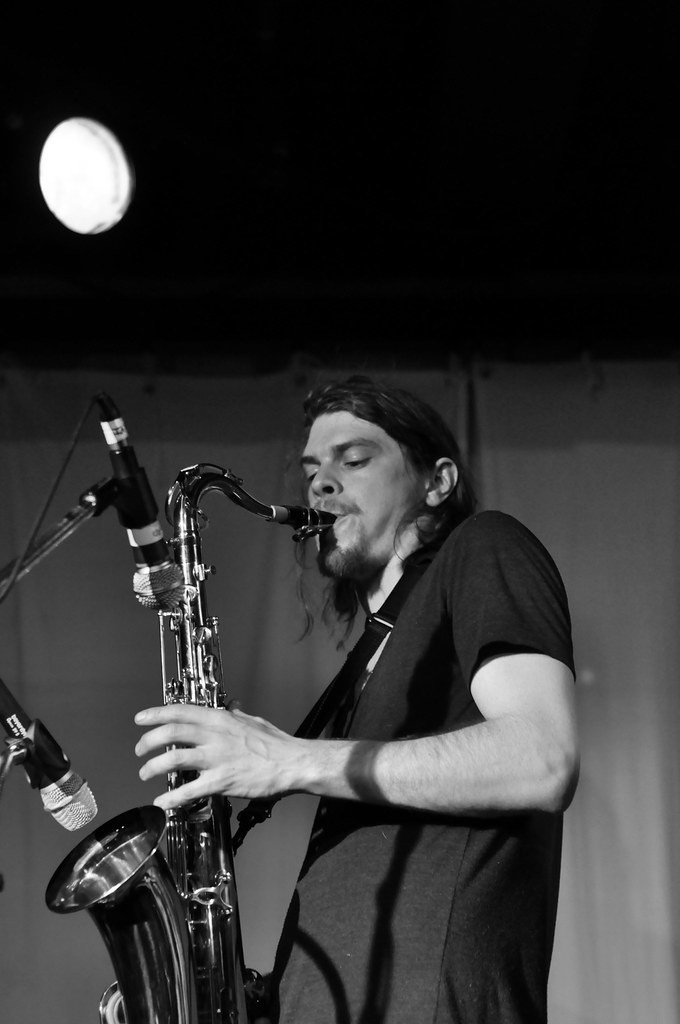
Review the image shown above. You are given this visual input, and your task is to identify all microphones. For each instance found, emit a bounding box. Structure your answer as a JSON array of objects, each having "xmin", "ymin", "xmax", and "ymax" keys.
[
  {"xmin": 96, "ymin": 392, "xmax": 186, "ymax": 611},
  {"xmin": 0, "ymin": 678, "xmax": 98, "ymax": 831}
]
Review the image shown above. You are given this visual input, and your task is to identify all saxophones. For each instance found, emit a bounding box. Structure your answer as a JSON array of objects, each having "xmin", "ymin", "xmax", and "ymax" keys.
[{"xmin": 44, "ymin": 460, "xmax": 337, "ymax": 1024}]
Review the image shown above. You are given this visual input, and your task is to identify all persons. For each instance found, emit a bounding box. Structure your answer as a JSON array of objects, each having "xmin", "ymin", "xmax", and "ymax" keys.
[{"xmin": 134, "ymin": 375, "xmax": 584, "ymax": 1024}]
